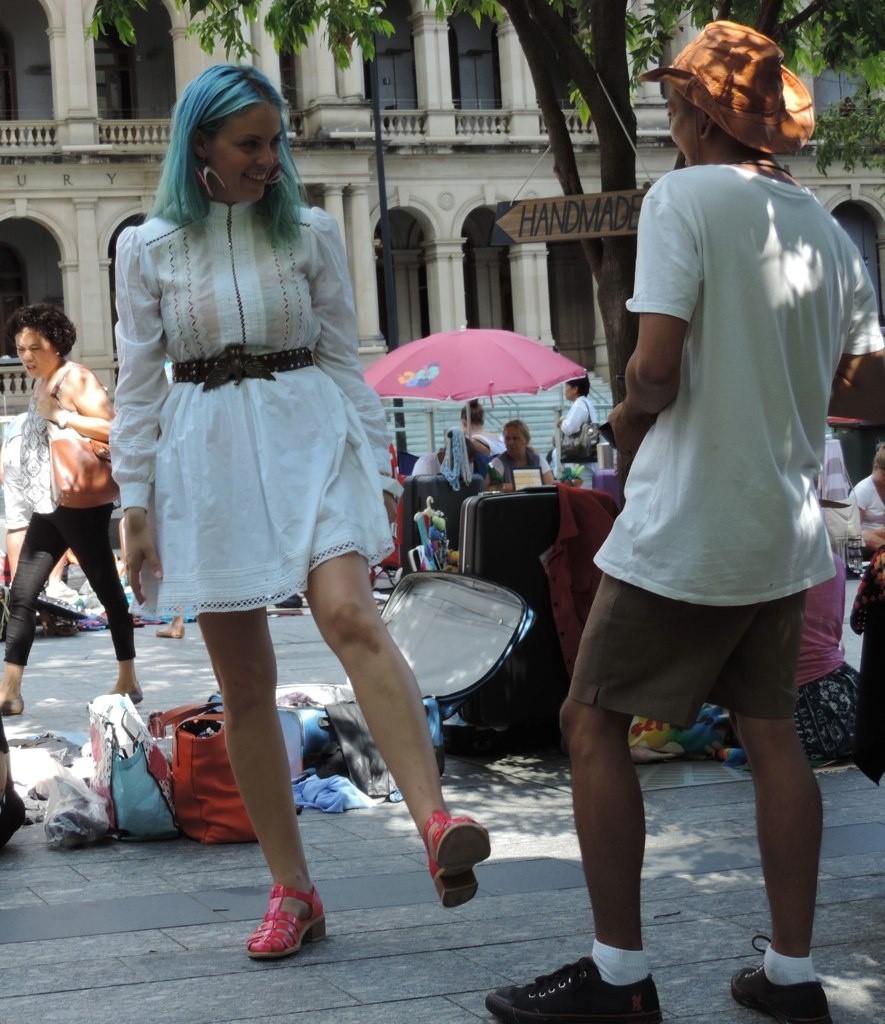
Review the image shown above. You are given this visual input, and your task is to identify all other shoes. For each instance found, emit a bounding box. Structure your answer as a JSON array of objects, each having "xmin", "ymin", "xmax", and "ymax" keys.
[
  {"xmin": 156, "ymin": 627, "xmax": 186, "ymax": 638},
  {"xmin": 266, "ymin": 593, "xmax": 302, "ymax": 614},
  {"xmin": 46, "ymin": 580, "xmax": 79, "ymax": 602}
]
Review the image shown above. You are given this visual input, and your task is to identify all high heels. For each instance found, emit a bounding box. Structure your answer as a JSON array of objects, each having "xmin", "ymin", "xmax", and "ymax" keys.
[
  {"xmin": 246, "ymin": 880, "xmax": 325, "ymax": 959},
  {"xmin": 423, "ymin": 809, "xmax": 492, "ymax": 909}
]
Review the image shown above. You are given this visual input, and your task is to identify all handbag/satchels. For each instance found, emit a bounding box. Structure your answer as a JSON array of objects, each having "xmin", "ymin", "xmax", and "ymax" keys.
[
  {"xmin": 88, "ymin": 692, "xmax": 181, "ymax": 843},
  {"xmin": 560, "ymin": 399, "xmax": 600, "ymax": 462},
  {"xmin": 160, "ymin": 699, "xmax": 264, "ymax": 845},
  {"xmin": 50, "ymin": 363, "xmax": 128, "ymax": 508},
  {"xmin": 814, "ymin": 439, "xmax": 866, "ymax": 548},
  {"xmin": 42, "ymin": 765, "xmax": 111, "ymax": 847}
]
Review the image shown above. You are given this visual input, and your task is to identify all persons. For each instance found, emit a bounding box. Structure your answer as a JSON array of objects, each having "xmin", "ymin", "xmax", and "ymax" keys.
[
  {"xmin": 0, "ymin": 300, "xmax": 885, "ymax": 852},
  {"xmin": 485, "ymin": 22, "xmax": 885, "ymax": 1024},
  {"xmin": 112, "ymin": 66, "xmax": 490, "ymax": 960}
]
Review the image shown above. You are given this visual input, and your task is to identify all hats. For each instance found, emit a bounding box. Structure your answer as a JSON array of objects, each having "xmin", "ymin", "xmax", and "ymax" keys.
[{"xmin": 638, "ymin": 20, "xmax": 815, "ymax": 155}]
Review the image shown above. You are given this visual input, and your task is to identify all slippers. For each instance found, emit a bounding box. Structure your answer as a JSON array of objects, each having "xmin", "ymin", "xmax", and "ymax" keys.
[
  {"xmin": 87, "ymin": 698, "xmax": 143, "ymax": 705},
  {"xmin": 1, "ymin": 709, "xmax": 22, "ymax": 714}
]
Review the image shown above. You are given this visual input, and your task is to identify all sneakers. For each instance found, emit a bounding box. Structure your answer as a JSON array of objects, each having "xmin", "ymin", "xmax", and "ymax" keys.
[
  {"xmin": 731, "ymin": 935, "xmax": 833, "ymax": 1024},
  {"xmin": 485, "ymin": 955, "xmax": 663, "ymax": 1024}
]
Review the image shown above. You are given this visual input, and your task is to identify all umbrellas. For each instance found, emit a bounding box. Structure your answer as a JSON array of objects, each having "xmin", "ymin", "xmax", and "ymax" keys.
[{"xmin": 363, "ymin": 326, "xmax": 586, "ymax": 444}]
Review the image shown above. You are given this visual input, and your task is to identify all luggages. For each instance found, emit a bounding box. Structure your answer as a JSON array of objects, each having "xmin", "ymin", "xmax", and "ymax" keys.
[
  {"xmin": 441, "ymin": 485, "xmax": 559, "ymax": 758},
  {"xmin": 398, "ymin": 430, "xmax": 485, "ymax": 581},
  {"xmin": 271, "ymin": 571, "xmax": 537, "ymax": 805}
]
